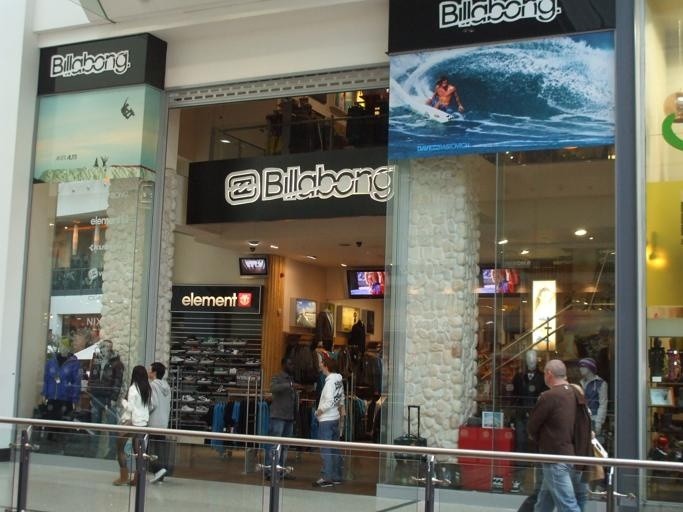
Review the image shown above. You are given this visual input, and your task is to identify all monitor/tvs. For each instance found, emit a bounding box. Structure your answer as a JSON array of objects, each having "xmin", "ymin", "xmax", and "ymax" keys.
[
  {"xmin": 478, "ymin": 266, "xmax": 521, "ymax": 298},
  {"xmin": 346, "ymin": 268, "xmax": 384, "ymax": 299},
  {"xmin": 239, "ymin": 257, "xmax": 267, "ymax": 279}
]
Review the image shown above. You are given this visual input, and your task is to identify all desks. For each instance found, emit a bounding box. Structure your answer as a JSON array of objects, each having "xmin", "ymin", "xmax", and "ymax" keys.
[{"xmin": 458, "ymin": 425, "xmax": 516, "ymax": 494}]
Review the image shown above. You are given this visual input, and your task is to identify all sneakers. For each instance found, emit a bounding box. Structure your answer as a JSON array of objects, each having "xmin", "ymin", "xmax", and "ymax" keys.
[
  {"xmin": 312, "ymin": 478, "xmax": 335, "ymax": 488},
  {"xmin": 148, "ymin": 465, "xmax": 168, "ymax": 483},
  {"xmin": 170, "ymin": 335, "xmax": 260, "ymax": 416},
  {"xmin": 491, "ymin": 475, "xmax": 504, "ymax": 494},
  {"xmin": 330, "ymin": 478, "xmax": 342, "ymax": 485},
  {"xmin": 509, "ymin": 478, "xmax": 523, "ymax": 493}
]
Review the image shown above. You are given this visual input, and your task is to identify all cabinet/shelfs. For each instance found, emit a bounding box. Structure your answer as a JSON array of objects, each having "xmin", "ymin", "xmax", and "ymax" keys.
[{"xmin": 168, "ymin": 336, "xmax": 260, "ymax": 420}]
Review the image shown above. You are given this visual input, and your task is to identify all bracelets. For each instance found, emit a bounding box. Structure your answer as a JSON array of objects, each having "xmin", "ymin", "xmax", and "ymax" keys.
[{"xmin": 290, "ymin": 381, "xmax": 293, "ymax": 388}]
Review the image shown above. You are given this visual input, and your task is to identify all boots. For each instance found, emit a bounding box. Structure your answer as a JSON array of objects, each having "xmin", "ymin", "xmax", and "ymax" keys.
[
  {"xmin": 658, "ymin": 411, "xmax": 682, "ymax": 433},
  {"xmin": 650, "ymin": 411, "xmax": 663, "ymax": 433},
  {"xmin": 113, "ymin": 466, "xmax": 129, "ymax": 486},
  {"xmin": 126, "ymin": 470, "xmax": 140, "ymax": 486}
]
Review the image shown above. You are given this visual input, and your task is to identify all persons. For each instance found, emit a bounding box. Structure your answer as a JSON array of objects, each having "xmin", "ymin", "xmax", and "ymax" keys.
[
  {"xmin": 263, "ymin": 356, "xmax": 304, "ymax": 482},
  {"xmin": 650, "ymin": 436, "xmax": 683, "ymax": 484},
  {"xmin": 39, "ymin": 337, "xmax": 81, "ymax": 442},
  {"xmin": 424, "ymin": 75, "xmax": 464, "ymax": 114},
  {"xmin": 571, "ymin": 356, "xmax": 608, "ymax": 492},
  {"xmin": 85, "ymin": 339, "xmax": 124, "ymax": 460},
  {"xmin": 524, "ymin": 358, "xmax": 587, "ymax": 512},
  {"xmin": 349, "ymin": 319, "xmax": 366, "ymax": 356},
  {"xmin": 364, "ymin": 271, "xmax": 383, "ymax": 296},
  {"xmin": 492, "ymin": 268, "xmax": 513, "ymax": 294},
  {"xmin": 314, "ymin": 308, "xmax": 335, "ymax": 353},
  {"xmin": 509, "ymin": 350, "xmax": 549, "ymax": 493},
  {"xmin": 306, "ymin": 348, "xmax": 336, "ymax": 453},
  {"xmin": 111, "ymin": 365, "xmax": 152, "ymax": 486},
  {"xmin": 311, "ymin": 357, "xmax": 345, "ymax": 488},
  {"xmin": 146, "ymin": 362, "xmax": 172, "ymax": 486}
]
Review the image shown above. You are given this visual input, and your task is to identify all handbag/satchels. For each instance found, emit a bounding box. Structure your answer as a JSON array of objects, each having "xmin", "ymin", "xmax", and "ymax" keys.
[
  {"xmin": 584, "ymin": 436, "xmax": 608, "ymax": 483},
  {"xmin": 116, "ymin": 410, "xmax": 132, "ymax": 438}
]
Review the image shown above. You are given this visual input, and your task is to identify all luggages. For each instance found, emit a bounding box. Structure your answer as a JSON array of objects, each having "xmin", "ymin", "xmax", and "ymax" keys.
[{"xmin": 393, "ymin": 403, "xmax": 428, "ymax": 478}]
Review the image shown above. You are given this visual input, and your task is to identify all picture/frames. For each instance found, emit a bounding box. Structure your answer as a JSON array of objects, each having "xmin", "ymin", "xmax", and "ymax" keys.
[
  {"xmin": 336, "ymin": 304, "xmax": 360, "ymax": 333},
  {"xmin": 295, "ymin": 297, "xmax": 317, "ymax": 329},
  {"xmin": 648, "ymin": 386, "xmax": 675, "ymax": 408},
  {"xmin": 482, "ymin": 411, "xmax": 504, "ymax": 430}
]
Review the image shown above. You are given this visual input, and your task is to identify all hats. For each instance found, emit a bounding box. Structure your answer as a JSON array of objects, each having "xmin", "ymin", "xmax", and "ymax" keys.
[{"xmin": 577, "ymin": 357, "xmax": 598, "ymax": 375}]
